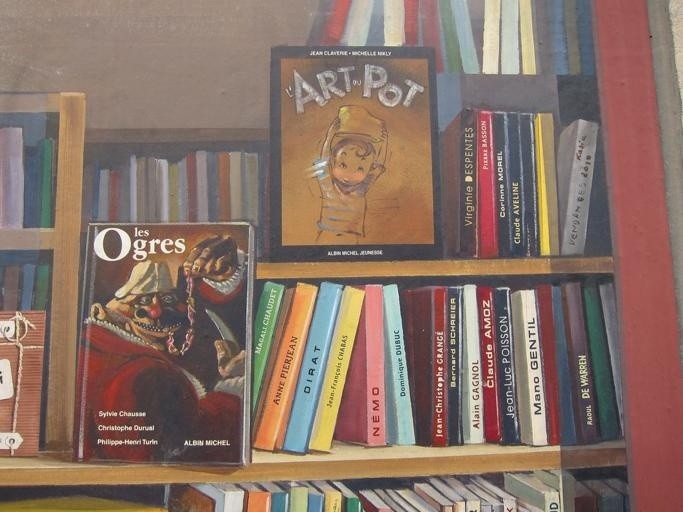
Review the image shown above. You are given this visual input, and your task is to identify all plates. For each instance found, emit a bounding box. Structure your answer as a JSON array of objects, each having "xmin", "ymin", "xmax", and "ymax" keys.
[{"xmin": 0, "ymin": 0, "xmax": 683, "ymax": 512}]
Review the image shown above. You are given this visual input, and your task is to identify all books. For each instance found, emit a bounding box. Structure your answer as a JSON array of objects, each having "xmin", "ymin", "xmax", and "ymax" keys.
[
  {"xmin": 3, "ymin": 264, "xmax": 51, "ymax": 457},
  {"xmin": 79, "ymin": 221, "xmax": 256, "ymax": 465},
  {"xmin": 439, "ymin": 107, "xmax": 600, "ymax": 258},
  {"xmin": 167, "ymin": 468, "xmax": 629, "ymax": 511},
  {"xmin": 251, "ymin": 280, "xmax": 625, "ymax": 456},
  {"xmin": 3, "ymin": 126, "xmax": 57, "ymax": 228},
  {"xmin": 270, "ymin": 45, "xmax": 443, "ymax": 262},
  {"xmin": 82, "ymin": 150, "xmax": 272, "ymax": 260}
]
